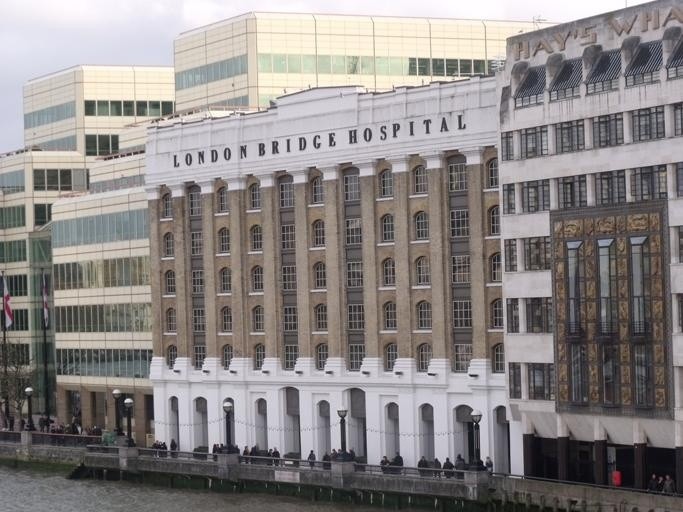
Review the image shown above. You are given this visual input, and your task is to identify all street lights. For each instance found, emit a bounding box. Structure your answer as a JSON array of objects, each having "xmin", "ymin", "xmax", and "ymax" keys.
[
  {"xmin": 23, "ymin": 387, "xmax": 37, "ymax": 431},
  {"xmin": 336, "ymin": 407, "xmax": 352, "ymax": 461},
  {"xmin": 469, "ymin": 411, "xmax": 486, "ymax": 471},
  {"xmin": 124, "ymin": 398, "xmax": 136, "ymax": 446},
  {"xmin": 223, "ymin": 402, "xmax": 235, "ymax": 453},
  {"xmin": 112, "ymin": 387, "xmax": 123, "ymax": 435}
]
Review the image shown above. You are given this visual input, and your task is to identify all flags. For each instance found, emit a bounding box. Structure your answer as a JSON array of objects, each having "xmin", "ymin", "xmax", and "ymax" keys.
[{"xmin": 42, "ymin": 271, "xmax": 49, "ymax": 328}]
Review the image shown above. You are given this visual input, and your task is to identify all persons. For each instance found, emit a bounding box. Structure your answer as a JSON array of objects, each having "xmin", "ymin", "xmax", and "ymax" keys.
[
  {"xmin": 322, "ymin": 448, "xmax": 355, "ymax": 470},
  {"xmin": 379, "ymin": 452, "xmax": 466, "ymax": 482},
  {"xmin": 211, "ymin": 443, "xmax": 280, "ymax": 467},
  {"xmin": 37, "ymin": 415, "xmax": 45, "ymax": 432},
  {"xmin": 484, "ymin": 457, "xmax": 493, "ymax": 475},
  {"xmin": 148, "ymin": 438, "xmax": 177, "ymax": 459},
  {"xmin": 49, "ymin": 422, "xmax": 103, "ymax": 447},
  {"xmin": 2, "ymin": 276, "xmax": 13, "ymax": 328},
  {"xmin": 307, "ymin": 450, "xmax": 315, "ymax": 468},
  {"xmin": 43, "ymin": 415, "xmax": 54, "ymax": 432},
  {"xmin": 644, "ymin": 472, "xmax": 677, "ymax": 497}
]
